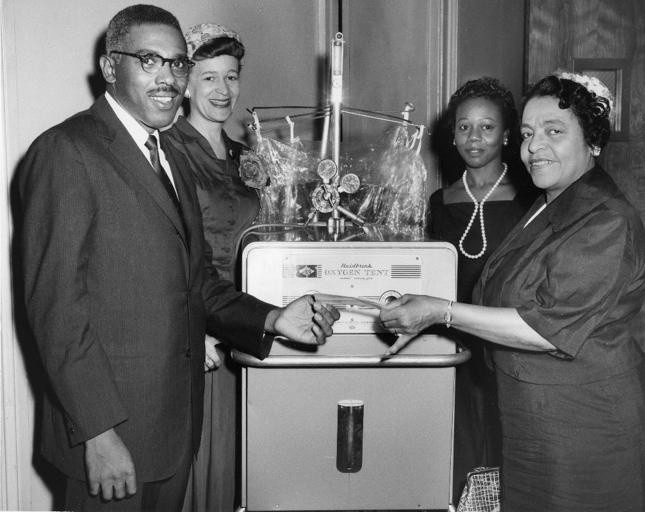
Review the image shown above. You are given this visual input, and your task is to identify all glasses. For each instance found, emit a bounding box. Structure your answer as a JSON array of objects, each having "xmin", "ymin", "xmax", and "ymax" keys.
[{"xmin": 108, "ymin": 50, "xmax": 196, "ymax": 75}]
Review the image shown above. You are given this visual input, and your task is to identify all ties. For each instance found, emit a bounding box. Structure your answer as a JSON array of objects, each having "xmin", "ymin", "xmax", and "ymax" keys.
[{"xmin": 144, "ymin": 135, "xmax": 183, "ymax": 217}]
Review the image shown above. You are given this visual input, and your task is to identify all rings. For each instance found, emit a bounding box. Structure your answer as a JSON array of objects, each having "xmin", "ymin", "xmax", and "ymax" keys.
[{"xmin": 395, "ymin": 329, "xmax": 400, "ymax": 337}]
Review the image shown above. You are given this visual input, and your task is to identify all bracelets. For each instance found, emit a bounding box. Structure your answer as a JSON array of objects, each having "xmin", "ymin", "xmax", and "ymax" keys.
[{"xmin": 445, "ymin": 301, "xmax": 454, "ymax": 330}]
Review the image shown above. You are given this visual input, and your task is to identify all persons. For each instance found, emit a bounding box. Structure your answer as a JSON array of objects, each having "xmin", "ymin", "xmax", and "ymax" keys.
[
  {"xmin": 426, "ymin": 81, "xmax": 543, "ymax": 504},
  {"xmin": 10, "ymin": 4, "xmax": 340, "ymax": 512},
  {"xmin": 161, "ymin": 22, "xmax": 271, "ymax": 512},
  {"xmin": 380, "ymin": 77, "xmax": 644, "ymax": 511}
]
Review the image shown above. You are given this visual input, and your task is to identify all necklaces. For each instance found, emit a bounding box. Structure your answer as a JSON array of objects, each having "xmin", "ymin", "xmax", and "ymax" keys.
[{"xmin": 458, "ymin": 162, "xmax": 508, "ymax": 260}]
